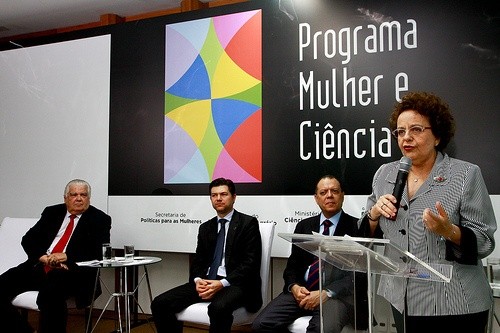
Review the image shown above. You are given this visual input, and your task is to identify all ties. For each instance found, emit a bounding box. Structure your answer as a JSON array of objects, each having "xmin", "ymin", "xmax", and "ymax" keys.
[
  {"xmin": 208, "ymin": 218, "xmax": 227, "ymax": 280},
  {"xmin": 306, "ymin": 219, "xmax": 334, "ymax": 291},
  {"xmin": 45, "ymin": 214, "xmax": 77, "ymax": 272}
]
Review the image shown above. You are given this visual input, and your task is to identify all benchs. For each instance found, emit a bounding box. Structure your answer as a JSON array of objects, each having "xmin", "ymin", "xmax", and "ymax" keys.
[{"xmin": 0, "ymin": 215, "xmax": 92, "ymax": 333}]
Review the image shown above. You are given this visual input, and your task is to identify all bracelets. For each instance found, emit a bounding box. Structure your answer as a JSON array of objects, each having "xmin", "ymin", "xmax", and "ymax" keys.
[
  {"xmin": 440, "ymin": 224, "xmax": 455, "ymax": 241},
  {"xmin": 366, "ymin": 208, "xmax": 379, "ymax": 221}
]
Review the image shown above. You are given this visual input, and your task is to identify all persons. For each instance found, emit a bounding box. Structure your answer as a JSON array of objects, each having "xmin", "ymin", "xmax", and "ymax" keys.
[
  {"xmin": 357, "ymin": 91, "xmax": 498, "ymax": 333},
  {"xmin": 0, "ymin": 179, "xmax": 112, "ymax": 333},
  {"xmin": 252, "ymin": 175, "xmax": 360, "ymax": 333},
  {"xmin": 150, "ymin": 178, "xmax": 263, "ymax": 333}
]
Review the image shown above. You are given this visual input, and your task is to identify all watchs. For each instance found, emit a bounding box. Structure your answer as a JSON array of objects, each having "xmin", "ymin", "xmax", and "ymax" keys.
[{"xmin": 326, "ymin": 291, "xmax": 332, "ymax": 298}]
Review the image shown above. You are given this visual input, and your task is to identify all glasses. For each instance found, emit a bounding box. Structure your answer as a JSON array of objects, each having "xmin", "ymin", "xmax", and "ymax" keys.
[{"xmin": 391, "ymin": 126, "xmax": 432, "ymax": 139}]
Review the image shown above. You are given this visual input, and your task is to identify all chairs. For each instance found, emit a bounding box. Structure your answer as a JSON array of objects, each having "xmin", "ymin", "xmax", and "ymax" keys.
[{"xmin": 176, "ymin": 221, "xmax": 275, "ymax": 333}]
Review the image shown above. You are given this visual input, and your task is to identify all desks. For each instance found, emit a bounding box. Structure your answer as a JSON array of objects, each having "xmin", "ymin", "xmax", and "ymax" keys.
[{"xmin": 89, "ymin": 256, "xmax": 162, "ymax": 333}]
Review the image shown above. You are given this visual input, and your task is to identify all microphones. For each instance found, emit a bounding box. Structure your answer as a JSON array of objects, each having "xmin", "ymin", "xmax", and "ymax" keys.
[{"xmin": 386, "ymin": 156, "xmax": 412, "ymax": 221}]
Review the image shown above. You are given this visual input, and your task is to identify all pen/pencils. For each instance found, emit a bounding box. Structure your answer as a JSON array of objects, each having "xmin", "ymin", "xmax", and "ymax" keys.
[{"xmin": 409, "ymin": 274, "xmax": 430, "ymax": 278}]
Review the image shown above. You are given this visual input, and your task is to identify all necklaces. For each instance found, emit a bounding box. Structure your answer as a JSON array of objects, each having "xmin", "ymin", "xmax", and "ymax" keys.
[{"xmin": 414, "ymin": 176, "xmax": 422, "ymax": 182}]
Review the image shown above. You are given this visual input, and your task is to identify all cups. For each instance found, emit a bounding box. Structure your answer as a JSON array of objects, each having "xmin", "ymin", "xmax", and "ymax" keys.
[
  {"xmin": 124, "ymin": 244, "xmax": 134, "ymax": 262},
  {"xmin": 487, "ymin": 258, "xmax": 500, "ymax": 289},
  {"xmin": 102, "ymin": 244, "xmax": 112, "ymax": 261}
]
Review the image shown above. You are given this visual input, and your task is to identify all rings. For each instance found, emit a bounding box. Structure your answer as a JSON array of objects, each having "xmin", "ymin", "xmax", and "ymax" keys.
[{"xmin": 381, "ymin": 204, "xmax": 385, "ymax": 209}]
